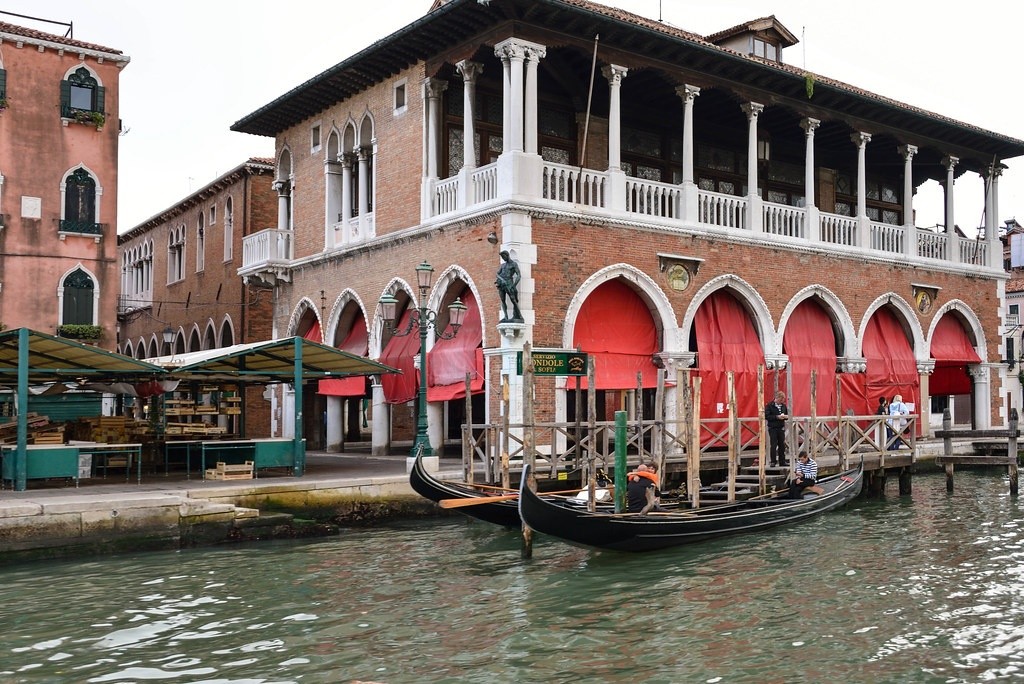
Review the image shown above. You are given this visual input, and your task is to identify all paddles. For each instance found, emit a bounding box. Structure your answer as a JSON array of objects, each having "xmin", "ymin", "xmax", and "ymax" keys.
[
  {"xmin": 437, "ymin": 486, "xmax": 614, "ymax": 511},
  {"xmin": 833, "ymin": 476, "xmax": 853, "ymax": 492},
  {"xmin": 443, "ymin": 478, "xmax": 520, "ymax": 492}
]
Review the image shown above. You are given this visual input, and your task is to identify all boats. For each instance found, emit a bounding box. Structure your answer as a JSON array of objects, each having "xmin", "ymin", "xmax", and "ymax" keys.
[
  {"xmin": 517, "ymin": 455, "xmax": 864, "ymax": 553},
  {"xmin": 408, "ymin": 444, "xmax": 616, "ymax": 527}
]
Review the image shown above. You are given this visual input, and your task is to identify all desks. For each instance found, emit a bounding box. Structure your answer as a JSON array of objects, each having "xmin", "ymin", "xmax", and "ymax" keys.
[
  {"xmin": 0, "ymin": 440, "xmax": 142, "ymax": 491},
  {"xmin": 166, "ymin": 436, "xmax": 306, "ymax": 480}
]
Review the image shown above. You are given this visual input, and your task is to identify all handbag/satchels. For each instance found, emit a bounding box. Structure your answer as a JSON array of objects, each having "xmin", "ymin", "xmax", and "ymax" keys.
[{"xmin": 900, "ymin": 418, "xmax": 908, "ymax": 426}]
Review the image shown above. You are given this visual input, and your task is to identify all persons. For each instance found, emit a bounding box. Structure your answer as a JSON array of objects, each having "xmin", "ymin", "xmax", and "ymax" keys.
[
  {"xmin": 495, "ymin": 251, "xmax": 521, "ymax": 320},
  {"xmin": 878, "ymin": 395, "xmax": 909, "ymax": 450},
  {"xmin": 765, "ymin": 392, "xmax": 790, "ymax": 467},
  {"xmin": 789, "ymin": 451, "xmax": 817, "ymax": 498},
  {"xmin": 627, "ymin": 462, "xmax": 668, "ymax": 515}
]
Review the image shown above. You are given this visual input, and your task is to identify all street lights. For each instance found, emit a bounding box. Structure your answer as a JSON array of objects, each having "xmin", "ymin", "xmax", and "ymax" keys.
[{"xmin": 377, "ymin": 259, "xmax": 467, "ymax": 456}]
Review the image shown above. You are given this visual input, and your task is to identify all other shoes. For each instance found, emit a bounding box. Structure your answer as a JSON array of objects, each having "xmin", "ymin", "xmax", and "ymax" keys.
[
  {"xmin": 779, "ymin": 462, "xmax": 789, "ymax": 466},
  {"xmin": 770, "ymin": 462, "xmax": 775, "ymax": 467}
]
